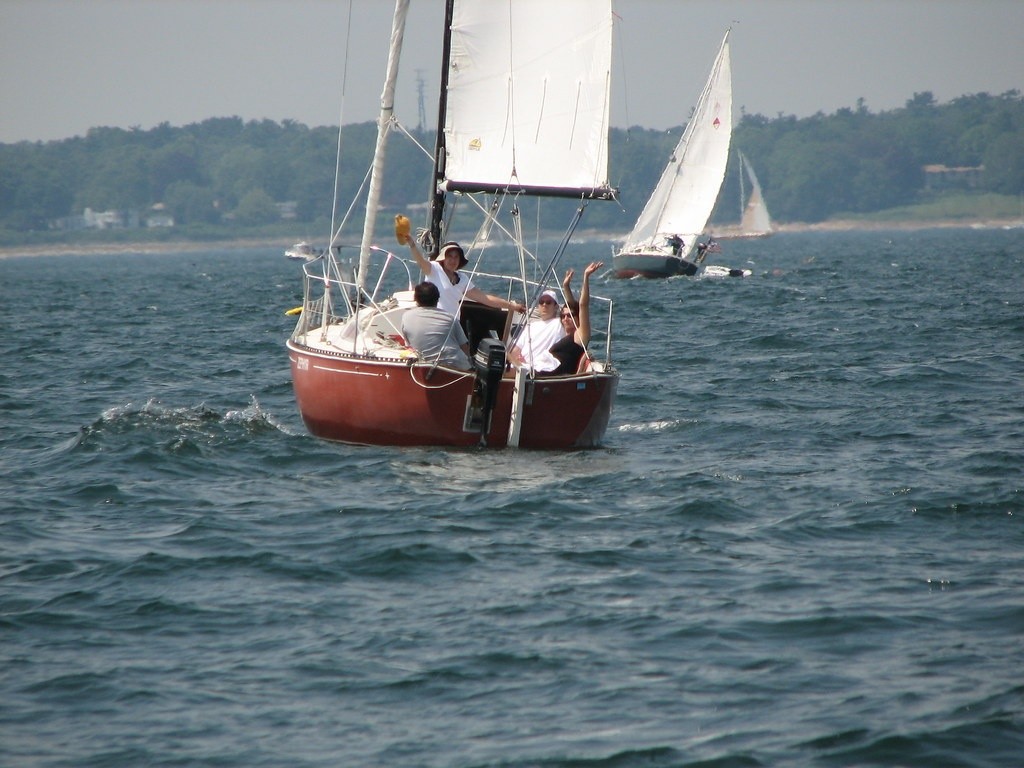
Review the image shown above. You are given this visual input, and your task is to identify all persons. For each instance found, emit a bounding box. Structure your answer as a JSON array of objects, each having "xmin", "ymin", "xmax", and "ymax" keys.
[
  {"xmin": 401, "ymin": 281, "xmax": 474, "ymax": 371},
  {"xmin": 668, "ymin": 234, "xmax": 685, "ymax": 258},
  {"xmin": 406, "ymin": 234, "xmax": 526, "ymax": 320},
  {"xmin": 507, "ymin": 261, "xmax": 603, "ymax": 379}
]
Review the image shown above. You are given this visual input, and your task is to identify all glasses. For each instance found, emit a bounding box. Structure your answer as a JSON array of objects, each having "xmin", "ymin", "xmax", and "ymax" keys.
[
  {"xmin": 560, "ymin": 312, "xmax": 577, "ymax": 319},
  {"xmin": 539, "ymin": 299, "xmax": 555, "ymax": 306}
]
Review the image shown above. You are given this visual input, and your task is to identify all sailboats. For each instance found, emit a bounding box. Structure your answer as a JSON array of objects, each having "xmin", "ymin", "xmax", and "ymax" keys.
[
  {"xmin": 285, "ymin": 0, "xmax": 620, "ymax": 450},
  {"xmin": 611, "ymin": 27, "xmax": 733, "ymax": 277},
  {"xmin": 734, "ymin": 149, "xmax": 774, "ymax": 238}
]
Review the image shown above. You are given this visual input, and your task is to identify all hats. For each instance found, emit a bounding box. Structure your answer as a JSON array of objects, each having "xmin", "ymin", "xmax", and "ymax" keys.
[
  {"xmin": 538, "ymin": 290, "xmax": 559, "ymax": 304},
  {"xmin": 394, "ymin": 214, "xmax": 410, "ymax": 246},
  {"xmin": 435, "ymin": 240, "xmax": 469, "ymax": 269}
]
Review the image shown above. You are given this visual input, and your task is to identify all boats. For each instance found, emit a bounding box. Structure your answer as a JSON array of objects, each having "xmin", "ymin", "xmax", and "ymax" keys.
[
  {"xmin": 285, "ymin": 241, "xmax": 316, "ymax": 262},
  {"xmin": 704, "ymin": 265, "xmax": 752, "ymax": 277}
]
